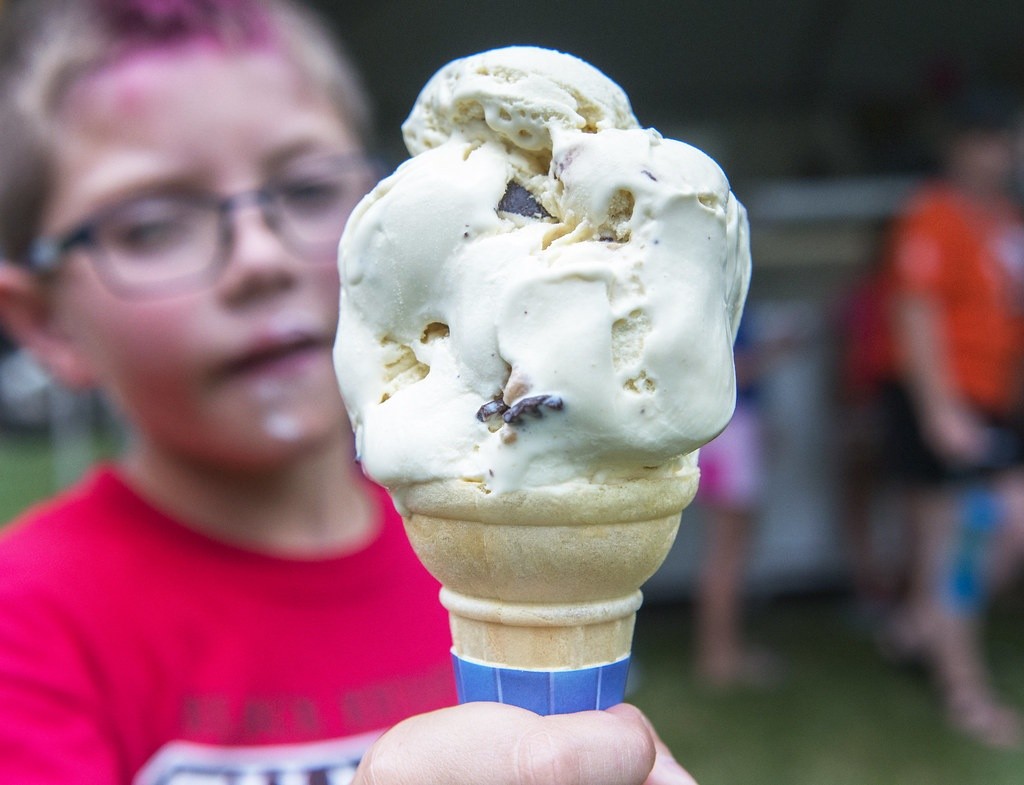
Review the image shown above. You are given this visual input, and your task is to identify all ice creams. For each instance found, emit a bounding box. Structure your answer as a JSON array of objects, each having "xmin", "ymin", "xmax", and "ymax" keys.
[{"xmin": 331, "ymin": 45, "xmax": 752, "ymax": 710}]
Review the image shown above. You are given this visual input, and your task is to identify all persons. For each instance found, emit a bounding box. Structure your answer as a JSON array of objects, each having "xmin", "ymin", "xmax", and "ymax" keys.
[
  {"xmin": 689, "ymin": 300, "xmax": 769, "ymax": 684},
  {"xmin": 0, "ymin": 1, "xmax": 704, "ymax": 785},
  {"xmin": 833, "ymin": 109, "xmax": 1023, "ymax": 762}
]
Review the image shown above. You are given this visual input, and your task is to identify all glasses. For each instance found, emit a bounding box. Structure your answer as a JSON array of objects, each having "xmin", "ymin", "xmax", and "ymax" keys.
[{"xmin": 28, "ymin": 162, "xmax": 395, "ymax": 295}]
